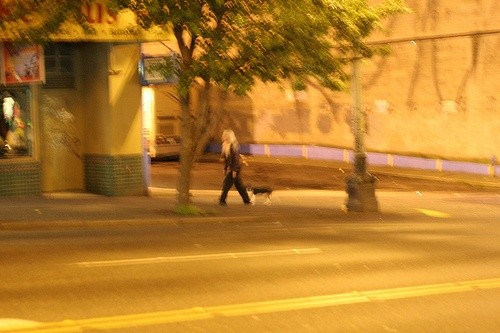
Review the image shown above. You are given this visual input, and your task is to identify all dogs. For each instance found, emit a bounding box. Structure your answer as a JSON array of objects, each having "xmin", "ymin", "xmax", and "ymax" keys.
[{"xmin": 245, "ymin": 185, "xmax": 273, "ymax": 206}]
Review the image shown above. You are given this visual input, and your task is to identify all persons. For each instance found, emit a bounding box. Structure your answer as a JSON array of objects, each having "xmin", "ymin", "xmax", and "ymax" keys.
[{"xmin": 218, "ymin": 129, "xmax": 255, "ymax": 206}]
